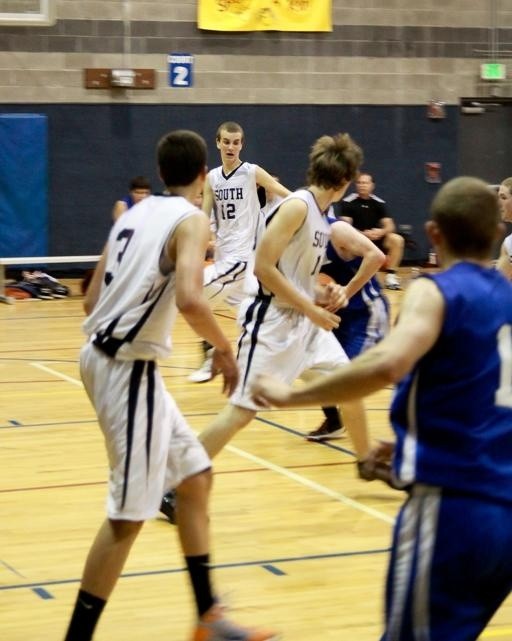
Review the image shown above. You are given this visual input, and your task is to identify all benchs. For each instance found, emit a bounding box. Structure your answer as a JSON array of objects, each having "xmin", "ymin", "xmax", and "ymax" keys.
[{"xmin": 0, "ymin": 254, "xmax": 102, "ymax": 306}]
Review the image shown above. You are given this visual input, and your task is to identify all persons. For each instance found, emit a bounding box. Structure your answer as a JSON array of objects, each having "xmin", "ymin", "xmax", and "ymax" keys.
[
  {"xmin": 195, "ymin": 188, "xmax": 219, "ymax": 261},
  {"xmin": 112, "ymin": 175, "xmax": 154, "ymax": 227},
  {"xmin": 247, "ymin": 175, "xmax": 511, "ymax": 641},
  {"xmin": 188, "ymin": 121, "xmax": 294, "ymax": 386},
  {"xmin": 494, "ymin": 179, "xmax": 512, "ymax": 286},
  {"xmin": 63, "ymin": 131, "xmax": 282, "ymax": 640},
  {"xmin": 155, "ymin": 133, "xmax": 393, "ymax": 527},
  {"xmin": 307, "ymin": 203, "xmax": 392, "ymax": 442},
  {"xmin": 339, "ymin": 171, "xmax": 406, "ymax": 291},
  {"xmin": 255, "ymin": 177, "xmax": 285, "ymax": 228}
]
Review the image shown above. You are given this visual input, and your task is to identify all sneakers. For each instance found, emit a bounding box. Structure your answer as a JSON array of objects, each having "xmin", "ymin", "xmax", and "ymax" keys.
[
  {"xmin": 192, "ymin": 603, "xmax": 276, "ymax": 641},
  {"xmin": 383, "ymin": 274, "xmax": 403, "ymax": 291},
  {"xmin": 306, "ymin": 418, "xmax": 346, "ymax": 442},
  {"xmin": 35, "ymin": 285, "xmax": 68, "ymax": 300},
  {"xmin": 187, "ymin": 345, "xmax": 222, "ymax": 383}
]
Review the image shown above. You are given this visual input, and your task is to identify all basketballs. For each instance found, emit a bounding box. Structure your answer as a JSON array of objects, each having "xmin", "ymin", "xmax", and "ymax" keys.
[{"xmin": 315, "ymin": 271, "xmax": 341, "ymax": 314}]
